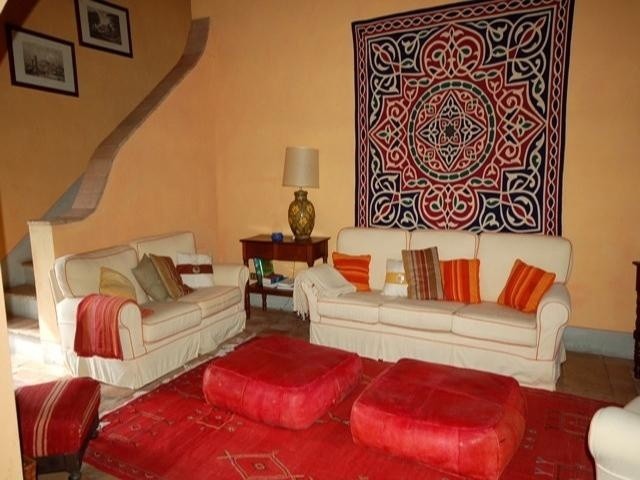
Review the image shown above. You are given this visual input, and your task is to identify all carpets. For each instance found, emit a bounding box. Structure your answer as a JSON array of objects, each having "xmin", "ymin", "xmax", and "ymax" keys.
[{"xmin": 78, "ymin": 330, "xmax": 624, "ymax": 480}]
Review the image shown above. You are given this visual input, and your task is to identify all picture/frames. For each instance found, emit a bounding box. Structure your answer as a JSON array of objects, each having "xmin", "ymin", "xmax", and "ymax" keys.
[
  {"xmin": 5, "ymin": 24, "xmax": 80, "ymax": 98},
  {"xmin": 73, "ymin": 0, "xmax": 134, "ymax": 58}
]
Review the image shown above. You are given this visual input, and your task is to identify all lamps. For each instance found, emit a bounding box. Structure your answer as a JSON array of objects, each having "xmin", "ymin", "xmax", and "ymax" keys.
[{"xmin": 281, "ymin": 146, "xmax": 320, "ymax": 240}]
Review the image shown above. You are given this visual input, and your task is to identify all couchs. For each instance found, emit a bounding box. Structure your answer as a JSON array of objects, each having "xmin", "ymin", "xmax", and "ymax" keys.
[
  {"xmin": 54, "ymin": 230, "xmax": 251, "ymax": 393},
  {"xmin": 300, "ymin": 226, "xmax": 573, "ymax": 390}
]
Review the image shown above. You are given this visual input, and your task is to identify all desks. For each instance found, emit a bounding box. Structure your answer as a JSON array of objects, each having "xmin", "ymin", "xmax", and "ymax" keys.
[
  {"xmin": 632, "ymin": 261, "xmax": 640, "ymax": 379},
  {"xmin": 238, "ymin": 233, "xmax": 330, "ymax": 318}
]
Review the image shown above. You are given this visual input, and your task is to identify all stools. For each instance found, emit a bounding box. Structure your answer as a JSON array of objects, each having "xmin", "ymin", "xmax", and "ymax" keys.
[
  {"xmin": 201, "ymin": 334, "xmax": 365, "ymax": 432},
  {"xmin": 348, "ymin": 356, "xmax": 529, "ymax": 480}
]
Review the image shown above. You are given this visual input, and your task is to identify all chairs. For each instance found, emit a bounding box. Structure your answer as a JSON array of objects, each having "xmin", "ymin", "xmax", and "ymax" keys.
[
  {"xmin": 586, "ymin": 396, "xmax": 640, "ymax": 480},
  {"xmin": 13, "ymin": 376, "xmax": 103, "ymax": 480}
]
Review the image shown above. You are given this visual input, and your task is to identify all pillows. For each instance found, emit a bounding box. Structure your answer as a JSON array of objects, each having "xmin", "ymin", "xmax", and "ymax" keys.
[
  {"xmin": 175, "ymin": 254, "xmax": 215, "ymax": 288},
  {"xmin": 130, "ymin": 252, "xmax": 169, "ymax": 304},
  {"xmin": 401, "ymin": 246, "xmax": 445, "ymax": 301},
  {"xmin": 440, "ymin": 259, "xmax": 483, "ymax": 305},
  {"xmin": 148, "ymin": 253, "xmax": 195, "ymax": 298},
  {"xmin": 332, "ymin": 251, "xmax": 372, "ymax": 292},
  {"xmin": 380, "ymin": 258, "xmax": 409, "ymax": 296},
  {"xmin": 98, "ymin": 267, "xmax": 138, "ymax": 303},
  {"xmin": 496, "ymin": 258, "xmax": 557, "ymax": 313}
]
